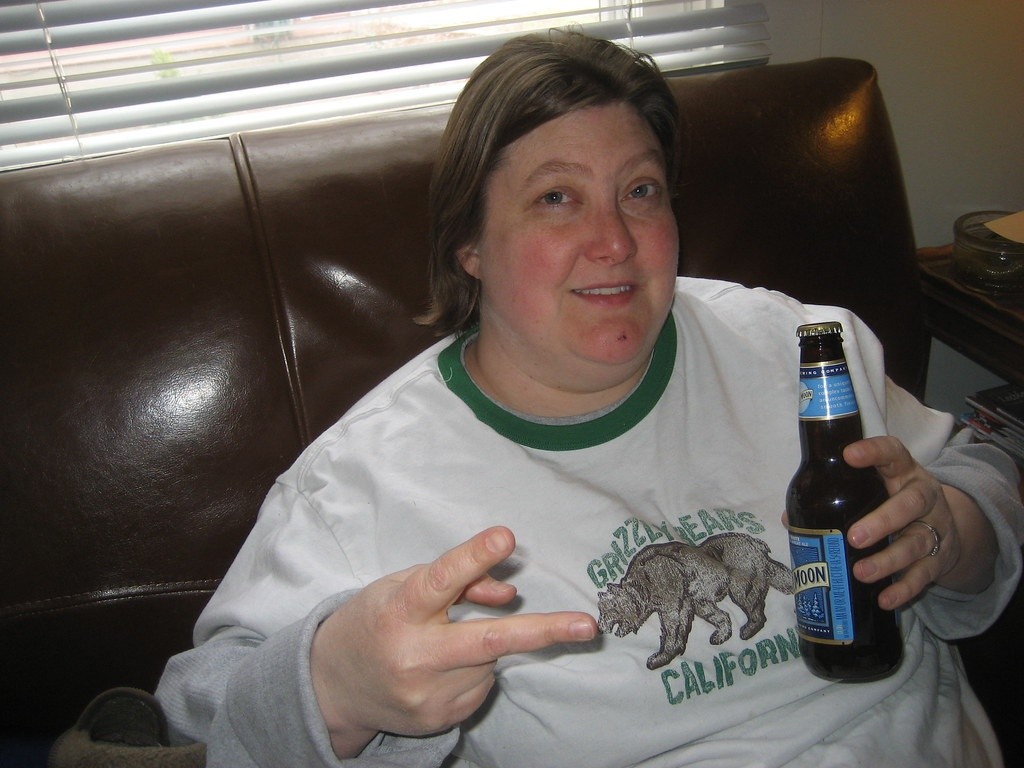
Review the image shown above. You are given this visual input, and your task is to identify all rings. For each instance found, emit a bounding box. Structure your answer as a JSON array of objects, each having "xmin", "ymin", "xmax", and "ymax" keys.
[{"xmin": 913, "ymin": 519, "xmax": 941, "ymax": 556}]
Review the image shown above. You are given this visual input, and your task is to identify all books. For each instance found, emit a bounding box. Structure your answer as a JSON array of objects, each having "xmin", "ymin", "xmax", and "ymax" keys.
[{"xmin": 959, "ymin": 381, "xmax": 1024, "ymax": 470}]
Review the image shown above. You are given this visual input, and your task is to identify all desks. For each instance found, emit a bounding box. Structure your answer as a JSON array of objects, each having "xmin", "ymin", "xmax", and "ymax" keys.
[{"xmin": 914, "ymin": 242, "xmax": 1024, "ymax": 391}]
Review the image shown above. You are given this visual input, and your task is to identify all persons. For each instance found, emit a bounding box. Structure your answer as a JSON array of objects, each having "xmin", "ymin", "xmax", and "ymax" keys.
[{"xmin": 158, "ymin": 30, "xmax": 1024, "ymax": 768}]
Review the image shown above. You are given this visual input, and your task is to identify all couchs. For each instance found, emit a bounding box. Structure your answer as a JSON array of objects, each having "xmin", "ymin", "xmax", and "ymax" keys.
[{"xmin": 0, "ymin": 57, "xmax": 1024, "ymax": 768}]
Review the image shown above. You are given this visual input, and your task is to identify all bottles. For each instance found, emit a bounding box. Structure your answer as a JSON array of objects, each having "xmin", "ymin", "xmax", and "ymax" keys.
[{"xmin": 784, "ymin": 326, "xmax": 905, "ymax": 683}]
